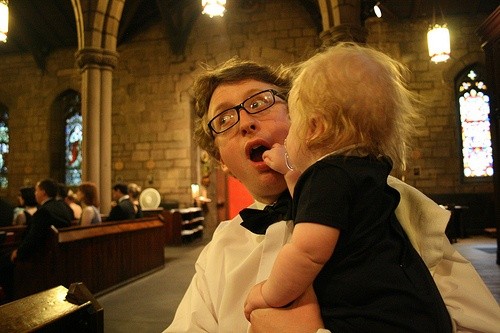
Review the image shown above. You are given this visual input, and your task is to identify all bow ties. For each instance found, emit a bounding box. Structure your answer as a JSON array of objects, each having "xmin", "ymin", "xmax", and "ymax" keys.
[{"xmin": 239, "ymin": 191, "xmax": 293, "ymax": 235}]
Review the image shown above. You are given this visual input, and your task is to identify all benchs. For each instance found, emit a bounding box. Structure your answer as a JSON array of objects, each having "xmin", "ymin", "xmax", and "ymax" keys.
[{"xmin": 0, "ymin": 213, "xmax": 167, "ymax": 300}]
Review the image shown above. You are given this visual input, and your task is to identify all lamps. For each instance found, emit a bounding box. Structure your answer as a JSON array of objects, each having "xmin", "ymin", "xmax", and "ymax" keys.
[{"xmin": 426, "ymin": 0, "xmax": 451, "ymax": 64}]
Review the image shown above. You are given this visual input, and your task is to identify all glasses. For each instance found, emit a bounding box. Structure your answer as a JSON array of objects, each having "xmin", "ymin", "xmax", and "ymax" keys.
[{"xmin": 208, "ymin": 89, "xmax": 288, "ymax": 139}]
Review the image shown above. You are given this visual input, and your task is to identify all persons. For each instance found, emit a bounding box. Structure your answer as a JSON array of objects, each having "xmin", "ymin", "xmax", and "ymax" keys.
[
  {"xmin": 76, "ymin": 181, "xmax": 103, "ymax": 225},
  {"xmin": 64, "ymin": 190, "xmax": 84, "ymax": 220},
  {"xmin": 126, "ymin": 184, "xmax": 145, "ymax": 219},
  {"xmin": 106, "ymin": 184, "xmax": 137, "ymax": 219},
  {"xmin": 161, "ymin": 59, "xmax": 500, "ymax": 333},
  {"xmin": 52, "ymin": 183, "xmax": 75, "ymax": 223},
  {"xmin": 13, "ymin": 186, "xmax": 39, "ymax": 225},
  {"xmin": 11, "ymin": 179, "xmax": 68, "ymax": 263},
  {"xmin": 243, "ymin": 40, "xmax": 454, "ymax": 333}
]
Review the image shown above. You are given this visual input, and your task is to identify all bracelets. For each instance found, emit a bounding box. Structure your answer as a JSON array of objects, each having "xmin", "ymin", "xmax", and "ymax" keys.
[{"xmin": 284, "ymin": 152, "xmax": 295, "ymax": 171}]
